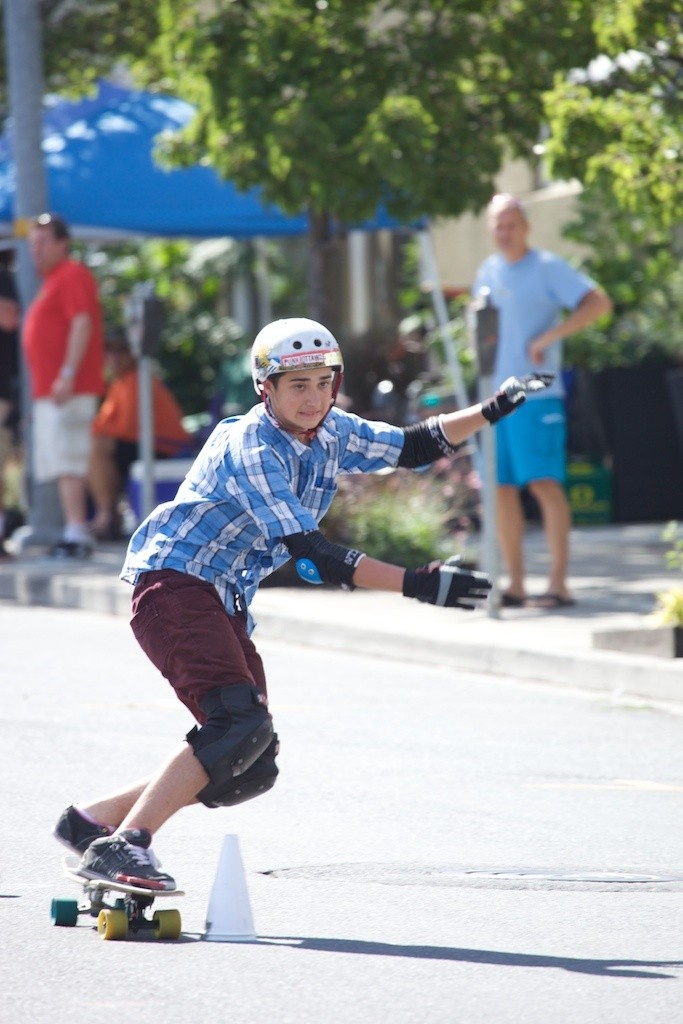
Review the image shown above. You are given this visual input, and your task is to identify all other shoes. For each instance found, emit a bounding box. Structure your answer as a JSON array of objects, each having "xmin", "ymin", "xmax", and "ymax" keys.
[{"xmin": 47, "ymin": 542, "xmax": 94, "ymax": 561}]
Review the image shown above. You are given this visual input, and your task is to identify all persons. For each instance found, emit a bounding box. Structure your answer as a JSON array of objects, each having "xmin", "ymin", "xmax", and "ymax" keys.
[
  {"xmin": 85, "ymin": 331, "xmax": 187, "ymax": 539},
  {"xmin": 0, "ymin": 268, "xmax": 18, "ymax": 484},
  {"xmin": 473, "ymin": 195, "xmax": 610, "ymax": 607},
  {"xmin": 23, "ymin": 215, "xmax": 105, "ymax": 560},
  {"xmin": 54, "ymin": 318, "xmax": 554, "ymax": 891}
]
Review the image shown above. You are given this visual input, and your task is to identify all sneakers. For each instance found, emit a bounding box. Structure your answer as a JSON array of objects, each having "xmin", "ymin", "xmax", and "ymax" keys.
[
  {"xmin": 53, "ymin": 802, "xmax": 118, "ymax": 857},
  {"xmin": 77, "ymin": 828, "xmax": 176, "ymax": 890}
]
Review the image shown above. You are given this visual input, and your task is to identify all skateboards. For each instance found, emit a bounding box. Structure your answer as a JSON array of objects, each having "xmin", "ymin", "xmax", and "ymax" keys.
[{"xmin": 51, "ymin": 853, "xmax": 187, "ymax": 939}]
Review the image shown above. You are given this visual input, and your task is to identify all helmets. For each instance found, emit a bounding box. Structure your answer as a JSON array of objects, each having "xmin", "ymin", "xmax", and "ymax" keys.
[{"xmin": 251, "ymin": 317, "xmax": 345, "ymax": 396}]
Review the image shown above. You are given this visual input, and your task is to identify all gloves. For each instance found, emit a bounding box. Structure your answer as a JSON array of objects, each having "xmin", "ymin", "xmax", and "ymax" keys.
[
  {"xmin": 482, "ymin": 371, "xmax": 555, "ymax": 424},
  {"xmin": 402, "ymin": 554, "xmax": 492, "ymax": 611}
]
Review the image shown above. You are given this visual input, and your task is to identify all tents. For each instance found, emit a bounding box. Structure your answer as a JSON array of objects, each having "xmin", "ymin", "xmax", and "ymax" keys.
[{"xmin": 0, "ymin": 86, "xmax": 482, "ymax": 512}]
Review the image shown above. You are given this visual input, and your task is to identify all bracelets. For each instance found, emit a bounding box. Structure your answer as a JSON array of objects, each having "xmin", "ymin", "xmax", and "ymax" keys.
[{"xmin": 61, "ymin": 368, "xmax": 74, "ymax": 377}]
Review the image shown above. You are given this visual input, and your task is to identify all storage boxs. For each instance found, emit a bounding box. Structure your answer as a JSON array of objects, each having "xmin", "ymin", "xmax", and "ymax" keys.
[{"xmin": 127, "ymin": 458, "xmax": 195, "ymax": 526}]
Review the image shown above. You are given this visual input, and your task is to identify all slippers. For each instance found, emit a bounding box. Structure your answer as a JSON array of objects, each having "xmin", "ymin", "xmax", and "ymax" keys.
[
  {"xmin": 500, "ymin": 591, "xmax": 525, "ymax": 606},
  {"xmin": 521, "ymin": 593, "xmax": 579, "ymax": 609}
]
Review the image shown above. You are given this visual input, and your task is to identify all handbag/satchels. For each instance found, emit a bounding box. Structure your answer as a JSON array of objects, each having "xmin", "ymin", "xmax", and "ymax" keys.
[{"xmin": 476, "ymin": 287, "xmax": 500, "ymax": 377}]
